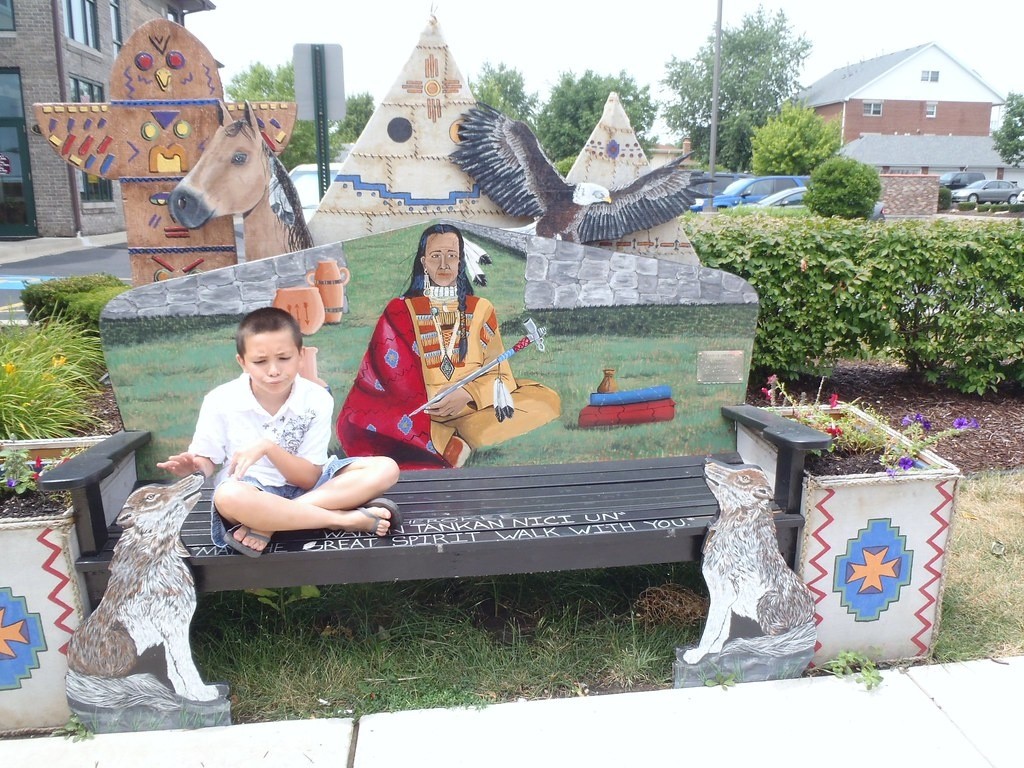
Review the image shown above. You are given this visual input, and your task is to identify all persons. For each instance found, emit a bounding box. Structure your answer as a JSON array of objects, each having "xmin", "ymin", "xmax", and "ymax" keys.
[{"xmin": 156, "ymin": 307, "xmax": 403, "ymax": 560}]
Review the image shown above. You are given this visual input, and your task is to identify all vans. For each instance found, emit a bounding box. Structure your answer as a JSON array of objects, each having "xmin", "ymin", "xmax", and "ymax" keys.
[{"xmin": 939, "ymin": 172, "xmax": 986, "ymax": 191}]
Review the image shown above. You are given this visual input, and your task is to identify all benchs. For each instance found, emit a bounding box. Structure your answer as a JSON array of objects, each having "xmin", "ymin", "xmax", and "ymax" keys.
[{"xmin": 35, "ymin": 219, "xmax": 832, "ymax": 646}]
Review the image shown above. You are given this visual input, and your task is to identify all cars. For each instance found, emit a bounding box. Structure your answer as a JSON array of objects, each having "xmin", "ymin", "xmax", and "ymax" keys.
[
  {"xmin": 702, "ymin": 173, "xmax": 751, "ymax": 195},
  {"xmin": 951, "ymin": 180, "xmax": 1023, "ymax": 205},
  {"xmin": 690, "ymin": 176, "xmax": 810, "ymax": 212},
  {"xmin": 738, "ymin": 186, "xmax": 884, "ymax": 221},
  {"xmin": 287, "ymin": 162, "xmax": 342, "ymax": 225},
  {"xmin": 1016, "ymin": 189, "xmax": 1024, "ymax": 204}
]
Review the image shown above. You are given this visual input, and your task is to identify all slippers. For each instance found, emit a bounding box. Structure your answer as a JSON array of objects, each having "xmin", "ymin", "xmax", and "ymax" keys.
[
  {"xmin": 222, "ymin": 524, "xmax": 271, "ymax": 558},
  {"xmin": 355, "ymin": 496, "xmax": 402, "ymax": 535}
]
